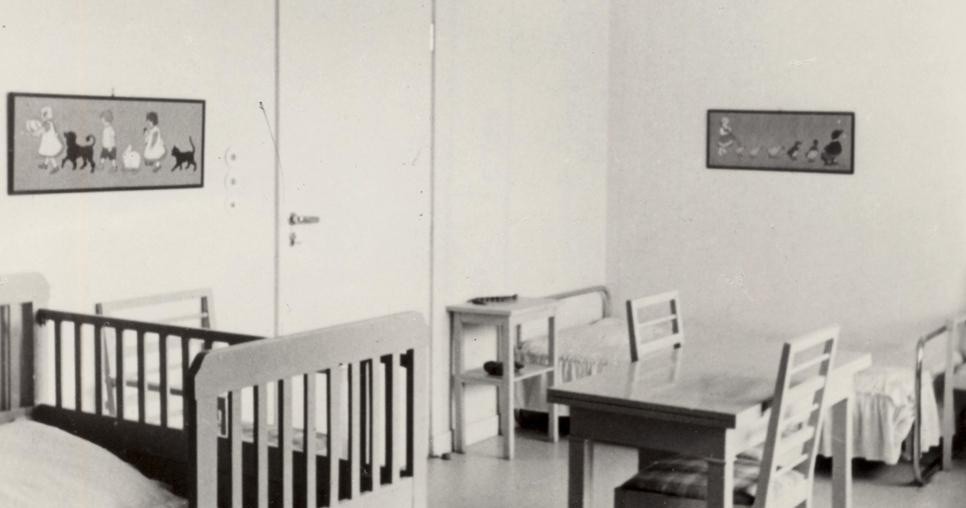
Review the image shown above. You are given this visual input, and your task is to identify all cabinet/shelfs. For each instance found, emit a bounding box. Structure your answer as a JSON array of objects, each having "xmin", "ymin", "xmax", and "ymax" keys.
[{"xmin": 445, "ymin": 292, "xmax": 560, "ymax": 462}]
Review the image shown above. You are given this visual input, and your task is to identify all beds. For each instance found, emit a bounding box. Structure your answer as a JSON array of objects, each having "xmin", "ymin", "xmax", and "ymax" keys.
[{"xmin": 510, "ymin": 283, "xmax": 965, "ymax": 484}]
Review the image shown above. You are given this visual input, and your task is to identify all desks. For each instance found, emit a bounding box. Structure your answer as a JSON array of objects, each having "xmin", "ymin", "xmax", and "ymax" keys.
[{"xmin": 542, "ymin": 332, "xmax": 874, "ymax": 507}]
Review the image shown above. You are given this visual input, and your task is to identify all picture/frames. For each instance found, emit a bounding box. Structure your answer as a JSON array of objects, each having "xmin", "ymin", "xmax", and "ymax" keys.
[
  {"xmin": 4, "ymin": 91, "xmax": 207, "ymax": 197},
  {"xmin": 704, "ymin": 106, "xmax": 856, "ymax": 176}
]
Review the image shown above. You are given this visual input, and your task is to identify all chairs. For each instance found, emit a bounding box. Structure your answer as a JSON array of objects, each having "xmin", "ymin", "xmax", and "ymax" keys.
[
  {"xmin": 611, "ymin": 318, "xmax": 840, "ymax": 507},
  {"xmin": 623, "ymin": 290, "xmax": 687, "ymax": 471}
]
